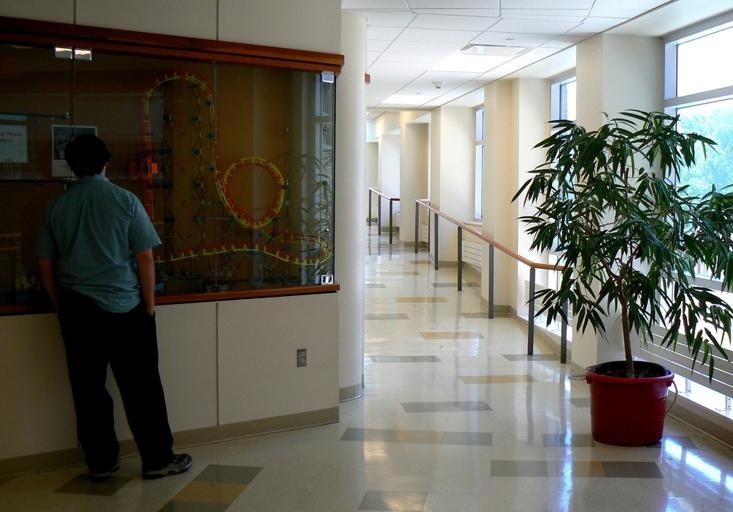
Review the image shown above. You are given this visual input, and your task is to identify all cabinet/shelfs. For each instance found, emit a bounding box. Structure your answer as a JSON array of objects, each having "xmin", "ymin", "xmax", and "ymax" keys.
[{"xmin": 216, "ymin": 290, "xmax": 340, "ymax": 428}]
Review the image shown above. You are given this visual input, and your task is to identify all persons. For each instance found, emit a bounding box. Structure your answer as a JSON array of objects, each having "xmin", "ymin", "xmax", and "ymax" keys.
[{"xmin": 39, "ymin": 133, "xmax": 193, "ymax": 480}]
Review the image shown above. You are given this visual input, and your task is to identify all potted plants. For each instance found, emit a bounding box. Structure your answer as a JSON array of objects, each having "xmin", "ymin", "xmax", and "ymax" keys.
[{"xmin": 509, "ymin": 107, "xmax": 733, "ymax": 447}]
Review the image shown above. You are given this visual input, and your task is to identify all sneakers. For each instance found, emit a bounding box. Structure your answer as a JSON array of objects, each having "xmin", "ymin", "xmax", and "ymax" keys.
[
  {"xmin": 142, "ymin": 453, "xmax": 192, "ymax": 479},
  {"xmin": 90, "ymin": 458, "xmax": 120, "ymax": 481}
]
